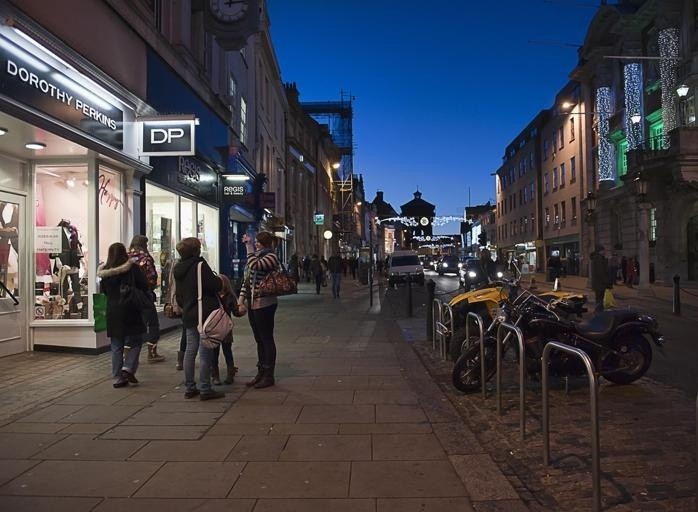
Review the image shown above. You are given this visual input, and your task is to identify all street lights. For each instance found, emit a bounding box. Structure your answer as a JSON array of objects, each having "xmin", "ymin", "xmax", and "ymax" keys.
[
  {"xmin": 631, "ymin": 110, "xmax": 643, "ymax": 147},
  {"xmin": 676, "ymin": 82, "xmax": 691, "ymax": 126}
]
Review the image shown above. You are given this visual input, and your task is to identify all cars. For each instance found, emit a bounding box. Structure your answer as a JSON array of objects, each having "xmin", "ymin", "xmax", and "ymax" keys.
[{"xmin": 418, "ymin": 253, "xmax": 503, "ymax": 292}]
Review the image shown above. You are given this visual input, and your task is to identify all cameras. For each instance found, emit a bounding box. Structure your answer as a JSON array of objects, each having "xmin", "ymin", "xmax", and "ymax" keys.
[{"xmin": 242, "ymin": 237, "xmax": 247, "ymax": 243}]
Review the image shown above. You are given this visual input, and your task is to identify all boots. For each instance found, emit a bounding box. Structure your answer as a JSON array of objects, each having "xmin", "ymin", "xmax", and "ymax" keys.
[
  {"xmin": 176, "ymin": 350, "xmax": 185, "ymax": 370},
  {"xmin": 254, "ymin": 365, "xmax": 274, "ymax": 388},
  {"xmin": 146, "ymin": 342, "xmax": 166, "ymax": 362},
  {"xmin": 246, "ymin": 361, "xmax": 264, "ymax": 387},
  {"xmin": 124, "ymin": 346, "xmax": 132, "ymax": 362},
  {"xmin": 224, "ymin": 367, "xmax": 238, "ymax": 384},
  {"xmin": 210, "ymin": 367, "xmax": 222, "ymax": 385}
]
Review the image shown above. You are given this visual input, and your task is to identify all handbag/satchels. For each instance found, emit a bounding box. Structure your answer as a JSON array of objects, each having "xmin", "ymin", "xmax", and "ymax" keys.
[
  {"xmin": 602, "ymin": 288, "xmax": 617, "ymax": 310},
  {"xmin": 119, "ymin": 283, "xmax": 152, "ymax": 312},
  {"xmin": 163, "ymin": 303, "xmax": 183, "ymax": 320},
  {"xmin": 197, "ymin": 308, "xmax": 233, "ymax": 350},
  {"xmin": 259, "ymin": 269, "xmax": 297, "ymax": 297},
  {"xmin": 321, "ymin": 278, "xmax": 328, "ymax": 287}
]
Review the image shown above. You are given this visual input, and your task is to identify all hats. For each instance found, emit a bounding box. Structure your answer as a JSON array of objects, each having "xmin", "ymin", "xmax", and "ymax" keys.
[{"xmin": 595, "ymin": 244, "xmax": 604, "ymax": 252}]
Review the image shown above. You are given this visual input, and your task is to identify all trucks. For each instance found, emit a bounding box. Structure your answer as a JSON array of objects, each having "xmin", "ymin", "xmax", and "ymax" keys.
[{"xmin": 388, "ymin": 249, "xmax": 426, "ymax": 289}]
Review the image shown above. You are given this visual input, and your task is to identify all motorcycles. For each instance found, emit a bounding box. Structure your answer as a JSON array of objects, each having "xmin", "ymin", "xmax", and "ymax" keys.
[
  {"xmin": 451, "ymin": 262, "xmax": 663, "ymax": 395},
  {"xmin": 446, "ymin": 277, "xmax": 586, "ymax": 358}
]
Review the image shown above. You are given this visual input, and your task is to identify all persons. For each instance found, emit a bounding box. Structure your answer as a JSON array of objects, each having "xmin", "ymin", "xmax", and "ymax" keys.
[
  {"xmin": 609, "ymin": 252, "xmax": 619, "ymax": 287},
  {"xmin": 475, "ymin": 248, "xmax": 497, "ymax": 288},
  {"xmin": 286, "ymin": 251, "xmax": 359, "ymax": 298},
  {"xmin": 620, "ymin": 255, "xmax": 630, "ymax": 285},
  {"xmin": 626, "ymin": 257, "xmax": 639, "ymax": 287},
  {"xmin": 547, "ymin": 249, "xmax": 561, "ymax": 293},
  {"xmin": 590, "ymin": 243, "xmax": 611, "ymax": 309},
  {"xmin": 49, "ymin": 218, "xmax": 85, "ymax": 318},
  {"xmin": 96, "ymin": 231, "xmax": 282, "ymax": 401}
]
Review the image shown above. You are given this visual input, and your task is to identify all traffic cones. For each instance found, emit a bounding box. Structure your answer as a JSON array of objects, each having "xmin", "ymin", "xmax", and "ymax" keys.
[{"xmin": 551, "ymin": 278, "xmax": 563, "ymax": 292}]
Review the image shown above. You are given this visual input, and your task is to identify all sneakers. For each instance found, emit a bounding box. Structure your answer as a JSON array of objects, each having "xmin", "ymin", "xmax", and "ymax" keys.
[
  {"xmin": 113, "ymin": 380, "xmax": 128, "ymax": 388},
  {"xmin": 199, "ymin": 389, "xmax": 225, "ymax": 401},
  {"xmin": 184, "ymin": 389, "xmax": 199, "ymax": 399},
  {"xmin": 121, "ymin": 367, "xmax": 138, "ymax": 383}
]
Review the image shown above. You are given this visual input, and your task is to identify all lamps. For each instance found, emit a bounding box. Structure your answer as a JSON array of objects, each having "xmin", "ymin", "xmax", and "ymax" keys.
[
  {"xmin": 633, "ymin": 172, "xmax": 647, "ymax": 202},
  {"xmin": 586, "ymin": 192, "xmax": 597, "ymax": 216},
  {"xmin": 222, "ymin": 174, "xmax": 249, "ymax": 180},
  {"xmin": 677, "ymin": 83, "xmax": 689, "ymax": 126},
  {"xmin": 632, "ymin": 110, "xmax": 641, "ymax": 147}
]
